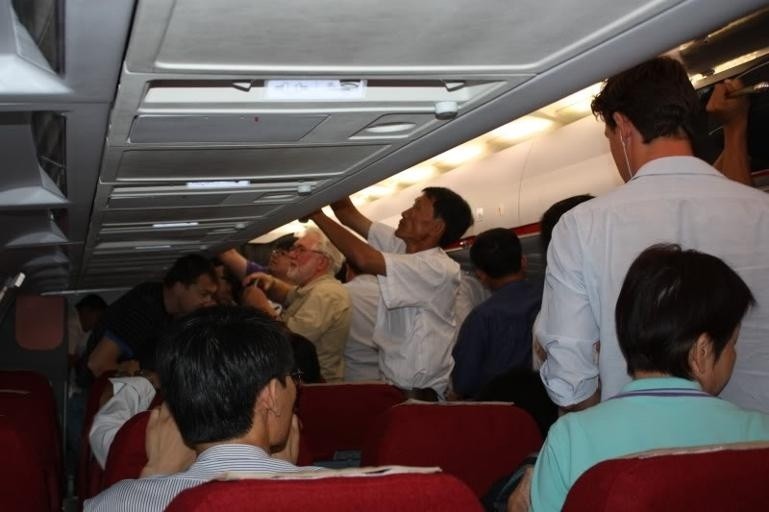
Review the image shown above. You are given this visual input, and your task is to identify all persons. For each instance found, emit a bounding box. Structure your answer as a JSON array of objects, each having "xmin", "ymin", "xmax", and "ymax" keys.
[
  {"xmin": 702, "ymin": 77, "xmax": 755, "ymax": 189},
  {"xmin": 506, "ymin": 240, "xmax": 769, "ymax": 512},
  {"xmin": 80, "ymin": 300, "xmax": 330, "ymax": 511},
  {"xmin": 531, "ymin": 53, "xmax": 769, "ymax": 418},
  {"xmin": 69, "ymin": 186, "xmax": 600, "ymax": 471}
]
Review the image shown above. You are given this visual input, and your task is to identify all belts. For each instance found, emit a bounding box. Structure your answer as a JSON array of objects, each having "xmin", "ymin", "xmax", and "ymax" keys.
[{"xmin": 397, "ymin": 387, "xmax": 438, "ymax": 402}]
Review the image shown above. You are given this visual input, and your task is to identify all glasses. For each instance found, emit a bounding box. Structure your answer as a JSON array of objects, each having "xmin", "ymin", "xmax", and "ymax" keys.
[
  {"xmin": 270, "ymin": 249, "xmax": 286, "ymax": 257},
  {"xmin": 288, "ymin": 245, "xmax": 323, "ymax": 255}
]
